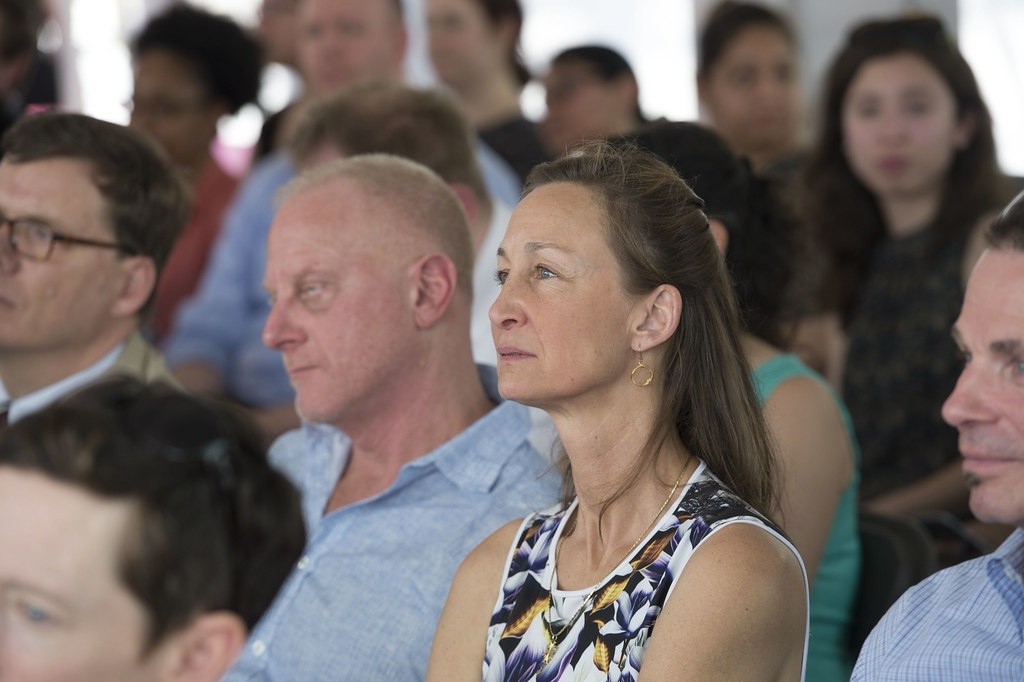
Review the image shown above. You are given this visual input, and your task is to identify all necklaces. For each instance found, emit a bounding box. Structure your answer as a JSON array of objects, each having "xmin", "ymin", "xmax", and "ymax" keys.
[{"xmin": 542, "ymin": 451, "xmax": 700, "ymax": 668}]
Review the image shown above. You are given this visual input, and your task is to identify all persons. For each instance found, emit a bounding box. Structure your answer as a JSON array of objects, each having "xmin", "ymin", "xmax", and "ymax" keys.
[
  {"xmin": 848, "ymin": 190, "xmax": 1022, "ymax": 680},
  {"xmin": 2, "ymin": 0, "xmax": 782, "ymax": 452},
  {"xmin": 200, "ymin": 153, "xmax": 569, "ymax": 682},
  {"xmin": 424, "ymin": 141, "xmax": 812, "ymax": 681},
  {"xmin": 687, "ymin": 0, "xmax": 846, "ymax": 405},
  {"xmin": 609, "ymin": 120, "xmax": 864, "ymax": 682},
  {"xmin": 1, "ymin": 373, "xmax": 304, "ymax": 682},
  {"xmin": 0, "ymin": 106, "xmax": 195, "ymax": 428},
  {"xmin": 811, "ymin": 15, "xmax": 1022, "ymax": 671}
]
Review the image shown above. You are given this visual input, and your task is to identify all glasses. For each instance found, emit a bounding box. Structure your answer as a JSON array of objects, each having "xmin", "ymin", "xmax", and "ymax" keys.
[
  {"xmin": 0, "ymin": 216, "xmax": 136, "ymax": 263},
  {"xmin": 122, "ymin": 97, "xmax": 185, "ymax": 119}
]
